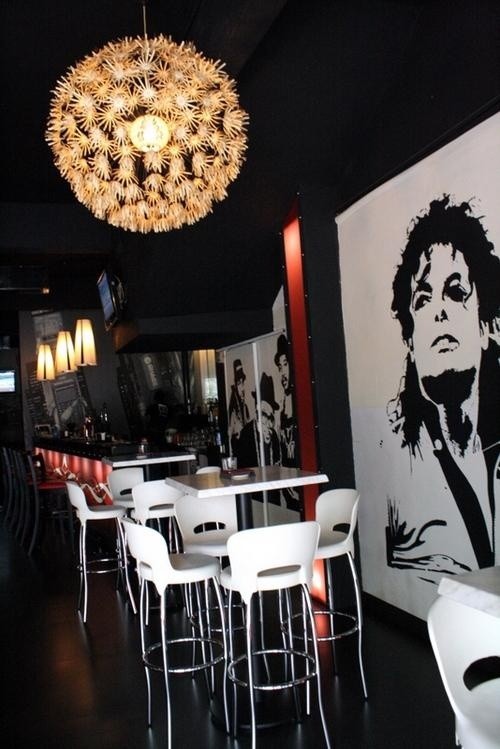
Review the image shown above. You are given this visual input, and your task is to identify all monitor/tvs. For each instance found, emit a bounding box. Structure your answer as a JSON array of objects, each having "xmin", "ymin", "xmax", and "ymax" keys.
[{"xmin": 96, "ymin": 269, "xmax": 127, "ymax": 333}]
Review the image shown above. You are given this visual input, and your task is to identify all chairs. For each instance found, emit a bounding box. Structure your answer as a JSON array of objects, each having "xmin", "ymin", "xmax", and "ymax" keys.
[
  {"xmin": 3, "ymin": 447, "xmax": 84, "ymax": 555},
  {"xmin": 131, "ymin": 479, "xmax": 202, "ymax": 626},
  {"xmin": 106, "ymin": 466, "xmax": 163, "ymax": 599},
  {"xmin": 63, "ymin": 481, "xmax": 141, "ymax": 624},
  {"xmin": 276, "ymin": 487, "xmax": 369, "ymax": 715},
  {"xmin": 217, "ymin": 521, "xmax": 332, "ymax": 748},
  {"xmin": 174, "ymin": 491, "xmax": 256, "ymax": 643},
  {"xmin": 426, "ymin": 594, "xmax": 500, "ymax": 749},
  {"xmin": 119, "ymin": 517, "xmax": 237, "ymax": 749}
]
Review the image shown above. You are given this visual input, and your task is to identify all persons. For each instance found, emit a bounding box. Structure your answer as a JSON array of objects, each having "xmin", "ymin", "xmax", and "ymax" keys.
[
  {"xmin": 275, "ymin": 335, "xmax": 302, "ymax": 513},
  {"xmin": 227, "ymin": 359, "xmax": 248, "ymax": 467},
  {"xmin": 236, "ymin": 372, "xmax": 279, "ymax": 506},
  {"xmin": 378, "ymin": 192, "xmax": 500, "ymax": 595}
]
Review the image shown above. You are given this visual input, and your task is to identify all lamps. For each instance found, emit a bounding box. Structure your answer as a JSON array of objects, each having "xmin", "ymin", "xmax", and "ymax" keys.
[
  {"xmin": 55, "ymin": 331, "xmax": 80, "ymax": 376},
  {"xmin": 36, "ymin": 340, "xmax": 57, "ymax": 383},
  {"xmin": 75, "ymin": 318, "xmax": 98, "ymax": 367},
  {"xmin": 42, "ymin": 32, "xmax": 251, "ymax": 234}
]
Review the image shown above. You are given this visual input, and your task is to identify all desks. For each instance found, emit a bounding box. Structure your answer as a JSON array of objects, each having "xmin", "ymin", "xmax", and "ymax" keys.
[
  {"xmin": 166, "ymin": 465, "xmax": 329, "ymax": 666},
  {"xmin": 34, "ymin": 437, "xmax": 143, "ymax": 525},
  {"xmin": 103, "ymin": 451, "xmax": 197, "ymax": 550}
]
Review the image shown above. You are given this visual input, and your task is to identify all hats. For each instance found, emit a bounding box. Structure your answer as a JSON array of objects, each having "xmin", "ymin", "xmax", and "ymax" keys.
[
  {"xmin": 252, "ymin": 372, "xmax": 280, "ymax": 410},
  {"xmin": 233, "ymin": 360, "xmax": 246, "ymax": 383},
  {"xmin": 275, "ymin": 335, "xmax": 289, "ymax": 365}
]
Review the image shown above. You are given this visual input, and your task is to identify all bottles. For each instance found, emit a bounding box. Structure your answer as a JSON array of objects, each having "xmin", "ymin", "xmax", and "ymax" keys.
[
  {"xmin": 97, "ymin": 407, "xmax": 109, "ymax": 442},
  {"xmin": 83, "ymin": 415, "xmax": 95, "ymax": 444}
]
